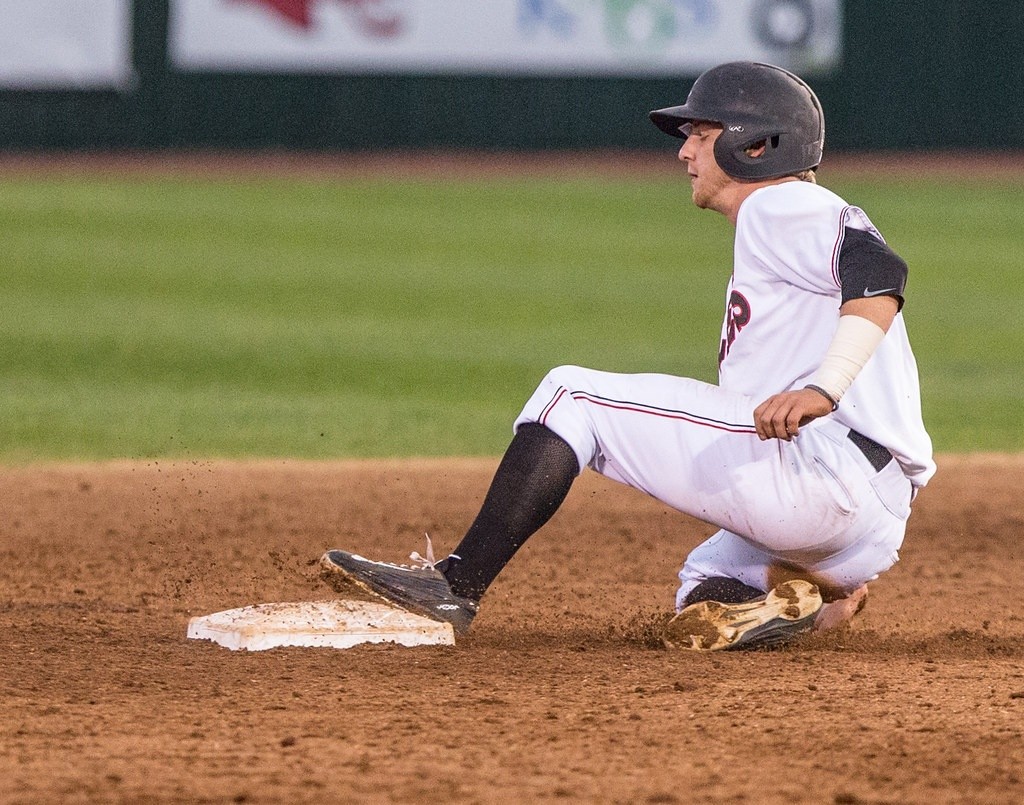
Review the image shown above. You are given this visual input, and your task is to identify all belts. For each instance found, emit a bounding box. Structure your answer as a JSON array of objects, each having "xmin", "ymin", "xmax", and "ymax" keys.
[{"xmin": 847, "ymin": 428, "xmax": 916, "ymax": 502}]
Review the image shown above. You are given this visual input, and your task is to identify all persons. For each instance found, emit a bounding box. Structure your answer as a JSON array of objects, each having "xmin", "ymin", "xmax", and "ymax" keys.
[{"xmin": 319, "ymin": 61, "xmax": 937, "ymax": 653}]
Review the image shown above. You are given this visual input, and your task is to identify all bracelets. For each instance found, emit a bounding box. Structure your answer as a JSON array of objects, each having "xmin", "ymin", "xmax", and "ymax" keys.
[{"xmin": 805, "ymin": 384, "xmax": 839, "ymax": 412}]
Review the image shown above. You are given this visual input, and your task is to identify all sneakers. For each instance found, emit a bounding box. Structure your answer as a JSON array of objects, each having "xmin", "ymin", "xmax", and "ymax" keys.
[
  {"xmin": 319, "ymin": 532, "xmax": 478, "ymax": 636},
  {"xmin": 662, "ymin": 580, "xmax": 822, "ymax": 652}
]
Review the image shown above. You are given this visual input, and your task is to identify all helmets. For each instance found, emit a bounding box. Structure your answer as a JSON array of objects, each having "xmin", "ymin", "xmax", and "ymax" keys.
[{"xmin": 649, "ymin": 60, "xmax": 827, "ymax": 180}]
